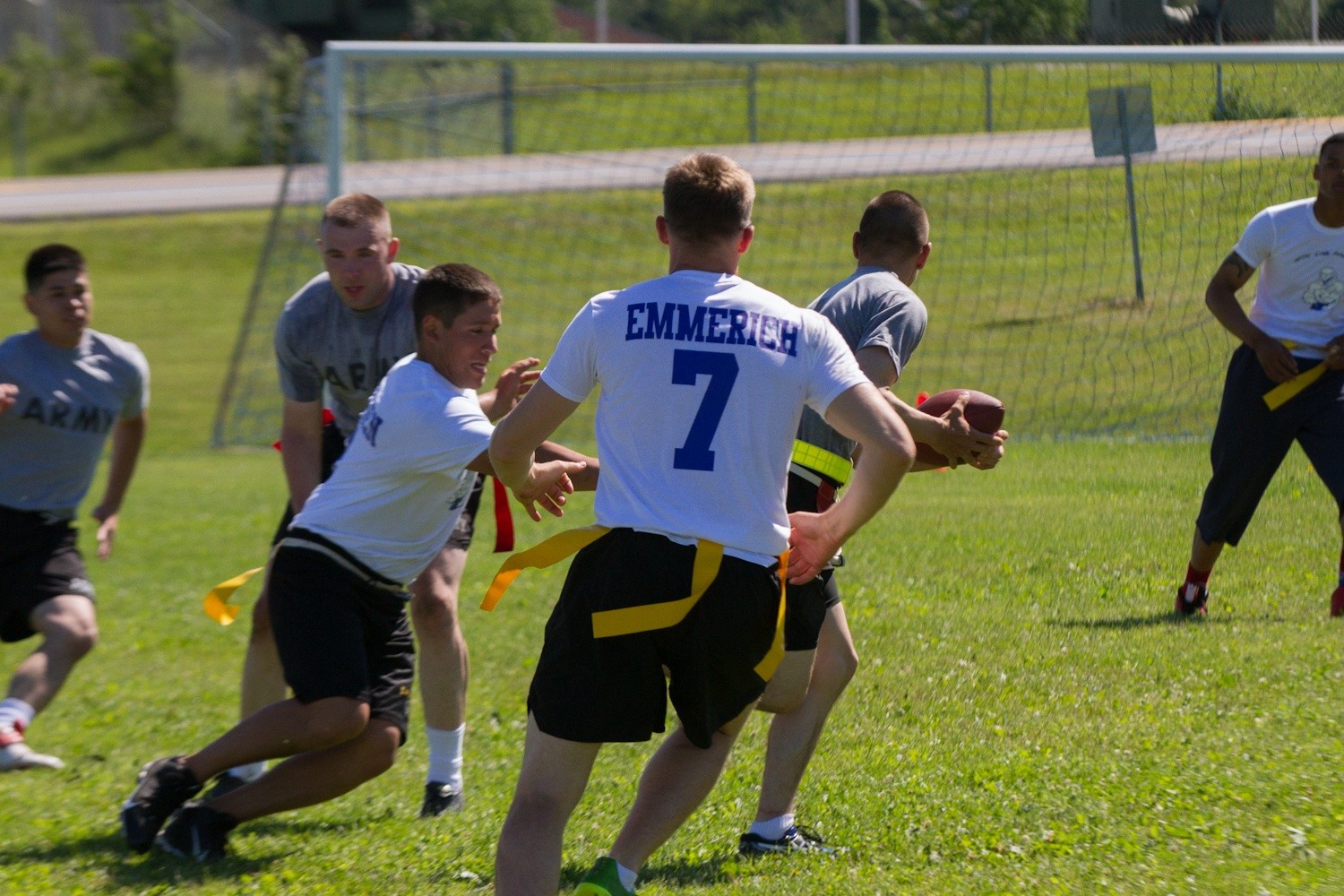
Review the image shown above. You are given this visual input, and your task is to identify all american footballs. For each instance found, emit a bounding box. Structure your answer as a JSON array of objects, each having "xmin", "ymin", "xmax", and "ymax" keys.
[{"xmin": 912, "ymin": 387, "xmax": 1007, "ymax": 468}]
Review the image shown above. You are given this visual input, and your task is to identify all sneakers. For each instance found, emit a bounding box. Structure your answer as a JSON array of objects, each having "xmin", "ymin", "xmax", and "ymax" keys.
[
  {"xmin": 202, "ymin": 772, "xmax": 247, "ymax": 805},
  {"xmin": 118, "ymin": 755, "xmax": 202, "ymax": 856},
  {"xmin": 1173, "ymin": 585, "xmax": 1210, "ymax": 623},
  {"xmin": 418, "ymin": 780, "xmax": 464, "ymax": 820},
  {"xmin": 570, "ymin": 855, "xmax": 639, "ymax": 896},
  {"xmin": 151, "ymin": 802, "xmax": 237, "ymax": 865},
  {"xmin": 0, "ymin": 724, "xmax": 65, "ymax": 773},
  {"xmin": 1329, "ymin": 584, "xmax": 1344, "ymax": 616},
  {"xmin": 734, "ymin": 824, "xmax": 849, "ymax": 862}
]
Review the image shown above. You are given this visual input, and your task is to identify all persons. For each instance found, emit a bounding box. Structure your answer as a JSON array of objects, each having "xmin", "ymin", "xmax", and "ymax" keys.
[
  {"xmin": 488, "ymin": 152, "xmax": 915, "ymax": 896},
  {"xmin": 192, "ymin": 195, "xmax": 485, "ymax": 822},
  {"xmin": 661, "ymin": 190, "xmax": 1010, "ymax": 851},
  {"xmin": 122, "ymin": 261, "xmax": 600, "ymax": 867},
  {"xmin": 1176, "ymin": 132, "xmax": 1344, "ymax": 616},
  {"xmin": 0, "ymin": 243, "xmax": 150, "ymax": 767}
]
